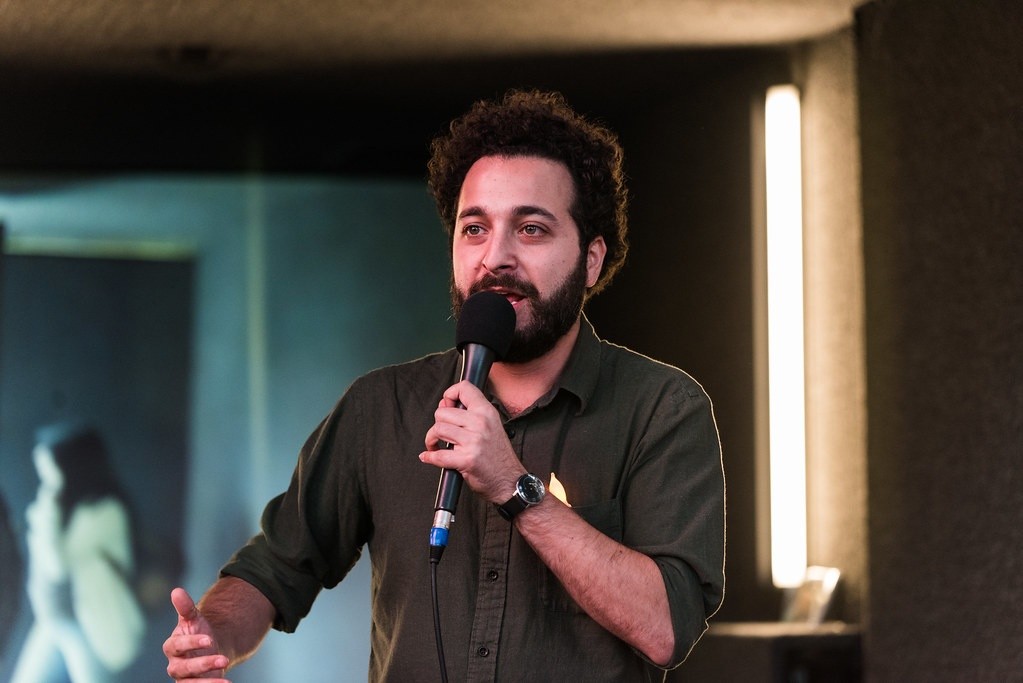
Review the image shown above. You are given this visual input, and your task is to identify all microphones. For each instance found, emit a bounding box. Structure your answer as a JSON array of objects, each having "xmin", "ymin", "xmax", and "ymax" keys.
[{"xmin": 428, "ymin": 290, "xmax": 517, "ymax": 563}]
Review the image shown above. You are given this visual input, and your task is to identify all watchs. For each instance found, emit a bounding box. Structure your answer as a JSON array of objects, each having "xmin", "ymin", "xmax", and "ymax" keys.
[{"xmin": 494, "ymin": 472, "xmax": 544, "ymax": 523}]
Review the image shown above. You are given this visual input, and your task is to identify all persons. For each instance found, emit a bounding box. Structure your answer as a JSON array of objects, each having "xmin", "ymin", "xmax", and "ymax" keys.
[
  {"xmin": 161, "ymin": 92, "xmax": 726, "ymax": 683},
  {"xmin": 8, "ymin": 420, "xmax": 147, "ymax": 683}
]
